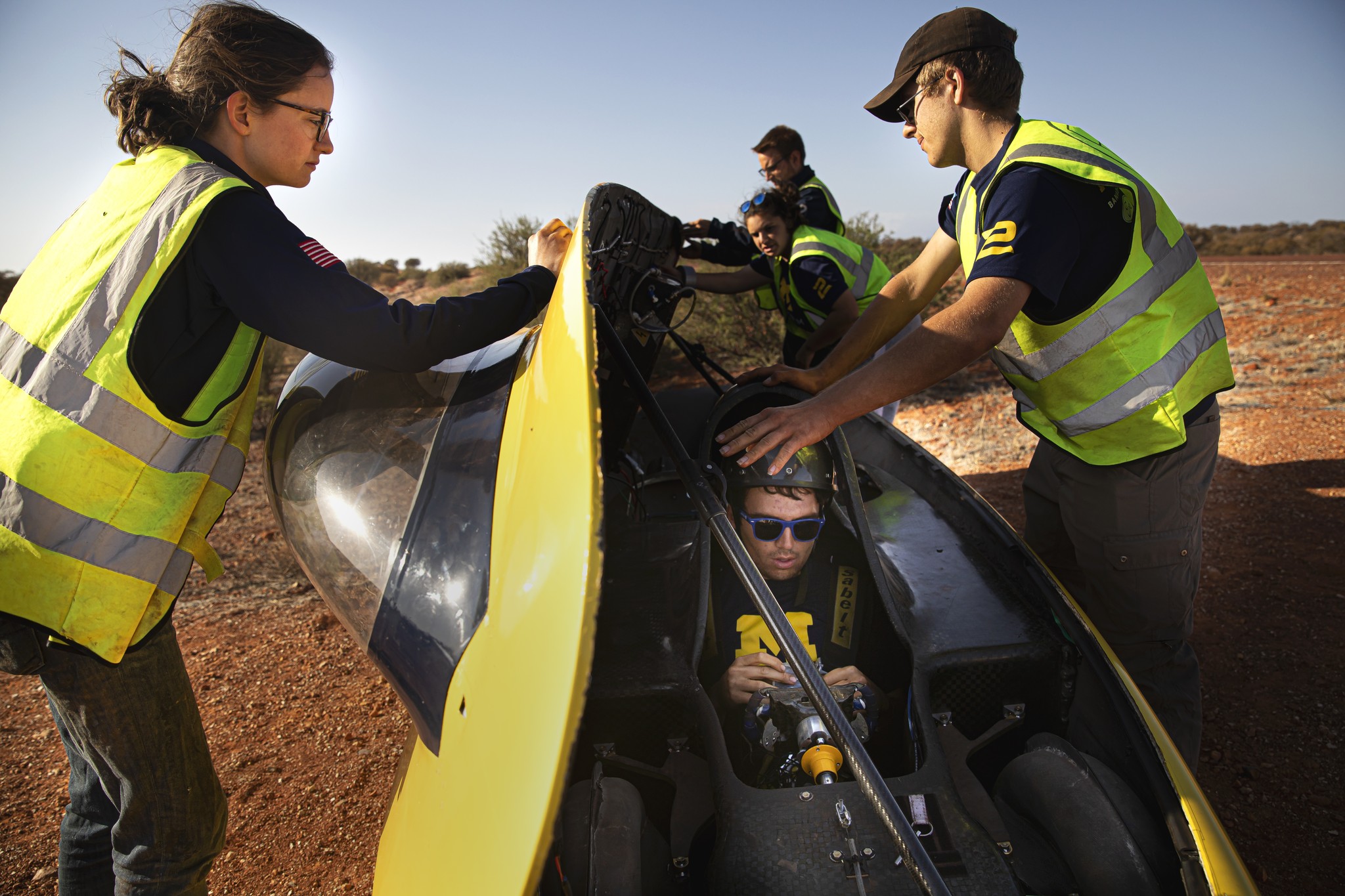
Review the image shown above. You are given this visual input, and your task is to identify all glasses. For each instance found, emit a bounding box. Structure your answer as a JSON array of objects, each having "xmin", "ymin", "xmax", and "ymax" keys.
[
  {"xmin": 896, "ymin": 72, "xmax": 971, "ymax": 125},
  {"xmin": 758, "ymin": 154, "xmax": 790, "ymax": 178},
  {"xmin": 739, "ymin": 192, "xmax": 770, "ymax": 213},
  {"xmin": 738, "ymin": 508, "xmax": 826, "ymax": 542},
  {"xmin": 218, "ymin": 92, "xmax": 334, "ymax": 142}
]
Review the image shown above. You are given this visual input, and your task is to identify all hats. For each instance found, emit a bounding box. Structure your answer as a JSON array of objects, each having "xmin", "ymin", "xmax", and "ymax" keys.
[{"xmin": 863, "ymin": 7, "xmax": 1015, "ymax": 123}]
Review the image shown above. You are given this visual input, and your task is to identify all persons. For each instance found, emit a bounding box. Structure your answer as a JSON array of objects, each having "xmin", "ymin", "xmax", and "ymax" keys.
[
  {"xmin": 1, "ymin": 0, "xmax": 572, "ymax": 896},
  {"xmin": 714, "ymin": 6, "xmax": 1235, "ymax": 774},
  {"xmin": 676, "ymin": 123, "xmax": 847, "ymax": 280},
  {"xmin": 653, "ymin": 187, "xmax": 923, "ymax": 434},
  {"xmin": 697, "ymin": 427, "xmax": 911, "ymax": 789}
]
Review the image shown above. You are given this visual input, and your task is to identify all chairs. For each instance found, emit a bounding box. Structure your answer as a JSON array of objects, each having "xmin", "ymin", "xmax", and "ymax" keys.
[{"xmin": 684, "ymin": 373, "xmax": 920, "ymax": 790}]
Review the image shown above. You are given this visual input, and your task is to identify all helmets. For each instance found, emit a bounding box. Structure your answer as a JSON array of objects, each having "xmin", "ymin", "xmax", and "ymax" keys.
[{"xmin": 722, "ymin": 438, "xmax": 838, "ymax": 493}]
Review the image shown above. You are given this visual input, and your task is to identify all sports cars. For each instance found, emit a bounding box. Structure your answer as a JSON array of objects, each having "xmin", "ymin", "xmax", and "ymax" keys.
[{"xmin": 260, "ymin": 181, "xmax": 1263, "ymax": 896}]
